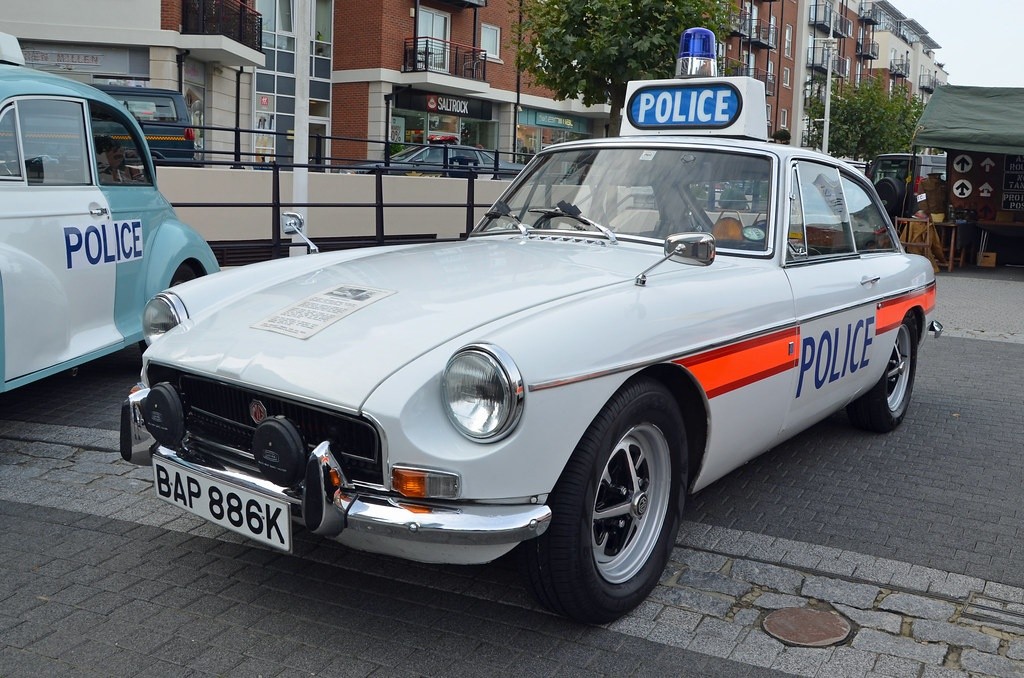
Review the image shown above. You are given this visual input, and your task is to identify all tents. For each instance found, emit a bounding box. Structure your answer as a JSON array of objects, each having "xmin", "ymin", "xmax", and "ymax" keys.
[{"xmin": 905, "ymin": 85, "xmax": 1024, "ymax": 253}]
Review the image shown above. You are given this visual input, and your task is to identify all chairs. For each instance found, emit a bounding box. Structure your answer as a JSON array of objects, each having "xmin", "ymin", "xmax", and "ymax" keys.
[
  {"xmin": 462, "ymin": 60, "xmax": 482, "ymax": 78},
  {"xmin": 895, "ymin": 216, "xmax": 930, "ymax": 259},
  {"xmin": 715, "ymin": 209, "xmax": 767, "ymax": 242}
]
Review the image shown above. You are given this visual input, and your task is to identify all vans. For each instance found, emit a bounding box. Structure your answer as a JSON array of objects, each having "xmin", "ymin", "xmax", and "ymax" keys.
[{"xmin": 0, "ymin": 83, "xmax": 196, "ymax": 167}]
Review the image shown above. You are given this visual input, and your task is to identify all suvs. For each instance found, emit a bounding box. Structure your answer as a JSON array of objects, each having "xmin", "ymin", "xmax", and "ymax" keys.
[{"xmin": 864, "ymin": 153, "xmax": 948, "ymax": 219}]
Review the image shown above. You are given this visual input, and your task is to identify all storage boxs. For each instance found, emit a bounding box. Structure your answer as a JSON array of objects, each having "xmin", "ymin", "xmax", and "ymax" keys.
[{"xmin": 976, "ymin": 252, "xmax": 997, "ymax": 266}]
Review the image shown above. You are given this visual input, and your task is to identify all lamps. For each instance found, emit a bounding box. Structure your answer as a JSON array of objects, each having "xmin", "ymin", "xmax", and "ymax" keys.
[{"xmin": 409, "ymin": 7, "xmax": 416, "ymax": 18}]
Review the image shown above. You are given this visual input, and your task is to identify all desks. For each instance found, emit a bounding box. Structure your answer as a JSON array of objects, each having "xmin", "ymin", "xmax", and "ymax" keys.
[
  {"xmin": 977, "ymin": 221, "xmax": 1024, "ymax": 266},
  {"xmin": 419, "ymin": 51, "xmax": 434, "ymax": 63},
  {"xmin": 932, "ymin": 223, "xmax": 966, "ymax": 272}
]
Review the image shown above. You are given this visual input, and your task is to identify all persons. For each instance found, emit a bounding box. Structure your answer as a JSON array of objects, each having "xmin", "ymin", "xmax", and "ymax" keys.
[
  {"xmin": 191, "ymin": 100, "xmax": 202, "ymax": 116},
  {"xmin": 773, "ymin": 130, "xmax": 792, "ymax": 145},
  {"xmin": 316, "ymin": 31, "xmax": 324, "ymax": 56},
  {"xmin": 476, "ymin": 144, "xmax": 483, "ymax": 149},
  {"xmin": 524, "ymin": 147, "xmax": 536, "ymax": 164},
  {"xmin": 95, "ymin": 135, "xmax": 125, "ymax": 185}
]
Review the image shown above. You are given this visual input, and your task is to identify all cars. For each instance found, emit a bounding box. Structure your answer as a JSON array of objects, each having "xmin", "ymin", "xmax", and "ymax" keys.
[
  {"xmin": 338, "ymin": 132, "xmax": 527, "ymax": 181},
  {"xmin": 688, "ymin": 178, "xmax": 769, "ymax": 195},
  {"xmin": 0, "ymin": 30, "xmax": 222, "ymax": 399},
  {"xmin": 119, "ymin": 27, "xmax": 950, "ymax": 629}
]
